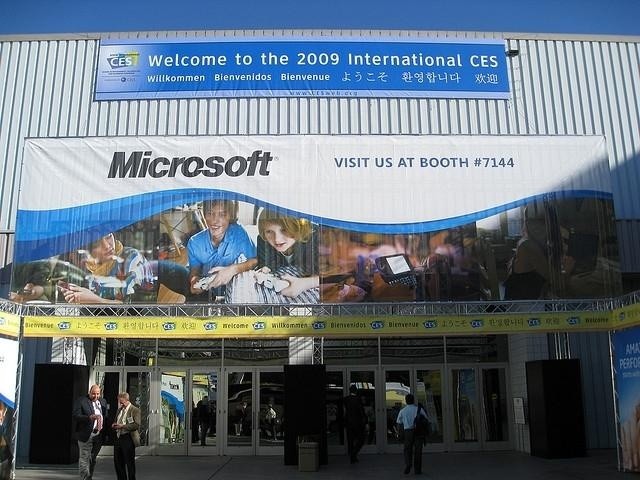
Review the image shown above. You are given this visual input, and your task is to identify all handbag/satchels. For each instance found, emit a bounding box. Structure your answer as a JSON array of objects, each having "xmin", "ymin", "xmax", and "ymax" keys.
[{"xmin": 412, "ymin": 406, "xmax": 432, "ymax": 447}]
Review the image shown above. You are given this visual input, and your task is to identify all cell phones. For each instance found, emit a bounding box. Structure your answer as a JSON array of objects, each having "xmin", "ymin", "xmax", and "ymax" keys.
[
  {"xmin": 376, "ymin": 254, "xmax": 419, "ymax": 291},
  {"xmin": 16, "ymin": 288, "xmax": 33, "ymax": 295},
  {"xmin": 58, "ymin": 278, "xmax": 70, "ymax": 290}
]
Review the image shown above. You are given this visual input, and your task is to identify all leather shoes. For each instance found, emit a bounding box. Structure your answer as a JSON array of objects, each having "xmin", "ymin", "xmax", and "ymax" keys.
[{"xmin": 403, "ymin": 464, "xmax": 412, "ymax": 475}]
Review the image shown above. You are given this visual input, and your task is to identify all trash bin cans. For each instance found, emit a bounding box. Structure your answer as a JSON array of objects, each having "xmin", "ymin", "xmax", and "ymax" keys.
[{"xmin": 296, "ymin": 434, "xmax": 321, "ymax": 472}]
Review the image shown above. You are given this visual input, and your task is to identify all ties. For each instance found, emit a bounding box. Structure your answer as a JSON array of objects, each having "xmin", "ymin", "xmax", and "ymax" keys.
[{"xmin": 116, "ymin": 410, "xmax": 125, "ymax": 439}]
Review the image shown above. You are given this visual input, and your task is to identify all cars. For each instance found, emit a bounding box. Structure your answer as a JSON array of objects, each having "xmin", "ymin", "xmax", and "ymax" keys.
[{"xmin": 228, "ymin": 386, "xmax": 282, "ymax": 420}]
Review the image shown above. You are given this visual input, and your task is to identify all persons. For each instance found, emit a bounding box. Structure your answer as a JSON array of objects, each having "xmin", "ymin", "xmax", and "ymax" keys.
[
  {"xmin": 72, "ymin": 384, "xmax": 107, "ymax": 480},
  {"xmin": 255, "ymin": 208, "xmax": 320, "ymax": 298},
  {"xmin": 111, "ymin": 392, "xmax": 141, "ymax": 480},
  {"xmin": 150, "ymin": 199, "xmax": 258, "ymax": 306},
  {"xmin": 192, "ymin": 396, "xmax": 276, "ymax": 446},
  {"xmin": 504, "ymin": 201, "xmax": 575, "ymax": 300},
  {"xmin": 343, "ymin": 384, "xmax": 432, "ymax": 475},
  {"xmin": 9, "ymin": 230, "xmax": 155, "ymax": 305},
  {"xmin": 0, "ymin": 400, "xmax": 13, "ymax": 480}
]
